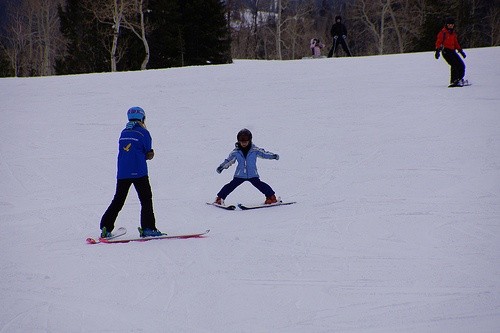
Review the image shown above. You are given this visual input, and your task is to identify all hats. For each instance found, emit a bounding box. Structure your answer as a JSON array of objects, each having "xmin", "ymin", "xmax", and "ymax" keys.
[{"xmin": 447, "ymin": 19, "xmax": 455, "ymax": 24}]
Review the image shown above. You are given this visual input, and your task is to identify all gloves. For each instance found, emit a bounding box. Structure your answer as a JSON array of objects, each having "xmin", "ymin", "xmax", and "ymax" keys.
[
  {"xmin": 216, "ymin": 166, "xmax": 223, "ymax": 174},
  {"xmin": 147, "ymin": 149, "xmax": 155, "ymax": 160},
  {"xmin": 458, "ymin": 49, "xmax": 466, "ymax": 58},
  {"xmin": 435, "ymin": 48, "xmax": 440, "ymax": 59},
  {"xmin": 342, "ymin": 35, "xmax": 347, "ymax": 38},
  {"xmin": 272, "ymin": 154, "xmax": 279, "ymax": 160},
  {"xmin": 334, "ymin": 36, "xmax": 338, "ymax": 39}
]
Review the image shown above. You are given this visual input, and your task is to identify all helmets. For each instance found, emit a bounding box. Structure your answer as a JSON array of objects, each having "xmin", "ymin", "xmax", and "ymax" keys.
[
  {"xmin": 127, "ymin": 106, "xmax": 145, "ymax": 124},
  {"xmin": 237, "ymin": 128, "xmax": 252, "ymax": 141}
]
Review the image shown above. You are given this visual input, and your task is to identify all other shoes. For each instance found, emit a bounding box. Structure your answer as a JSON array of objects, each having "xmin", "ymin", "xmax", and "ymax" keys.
[{"xmin": 453, "ymin": 80, "xmax": 461, "ymax": 86}]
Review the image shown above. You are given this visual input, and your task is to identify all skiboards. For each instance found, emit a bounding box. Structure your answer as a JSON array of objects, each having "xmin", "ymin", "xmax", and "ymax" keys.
[
  {"xmin": 84, "ymin": 226, "xmax": 211, "ymax": 244},
  {"xmin": 206, "ymin": 196, "xmax": 282, "ymax": 210},
  {"xmin": 448, "ymin": 78, "xmax": 472, "ymax": 87}
]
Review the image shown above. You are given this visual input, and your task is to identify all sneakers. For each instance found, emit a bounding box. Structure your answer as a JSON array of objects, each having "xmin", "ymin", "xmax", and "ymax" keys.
[
  {"xmin": 215, "ymin": 196, "xmax": 225, "ymax": 207},
  {"xmin": 265, "ymin": 194, "xmax": 278, "ymax": 204},
  {"xmin": 138, "ymin": 227, "xmax": 167, "ymax": 238},
  {"xmin": 101, "ymin": 227, "xmax": 114, "ymax": 238}
]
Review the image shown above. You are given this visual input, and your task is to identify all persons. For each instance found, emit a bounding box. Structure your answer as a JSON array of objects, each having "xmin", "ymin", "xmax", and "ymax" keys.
[
  {"xmin": 214, "ymin": 128, "xmax": 279, "ymax": 205},
  {"xmin": 310, "ymin": 38, "xmax": 325, "ymax": 56},
  {"xmin": 99, "ymin": 107, "xmax": 168, "ymax": 237},
  {"xmin": 327, "ymin": 16, "xmax": 353, "ymax": 57},
  {"xmin": 435, "ymin": 18, "xmax": 467, "ymax": 84}
]
